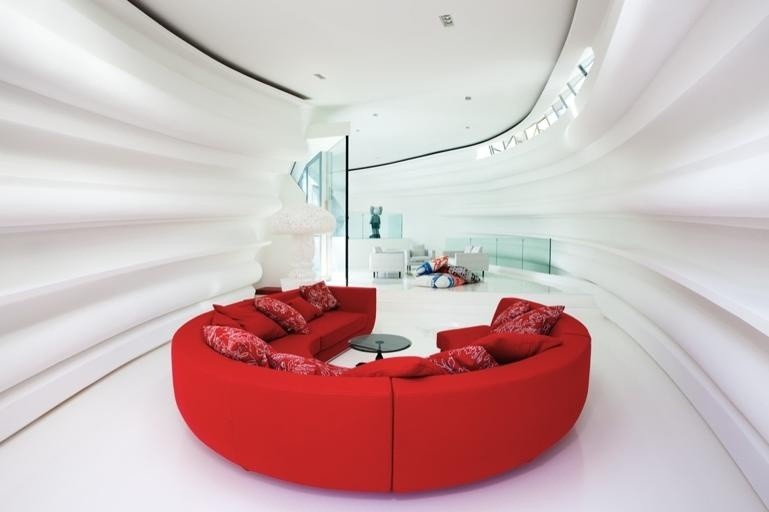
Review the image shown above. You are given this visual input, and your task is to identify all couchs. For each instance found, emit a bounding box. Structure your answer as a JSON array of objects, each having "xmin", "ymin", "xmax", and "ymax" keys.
[
  {"xmin": 435, "ymin": 295, "xmax": 592, "ymax": 349},
  {"xmin": 391, "ymin": 333, "xmax": 592, "ymax": 495},
  {"xmin": 168, "ymin": 300, "xmax": 393, "ymax": 497},
  {"xmin": 250, "ymin": 283, "xmax": 377, "ymax": 363}
]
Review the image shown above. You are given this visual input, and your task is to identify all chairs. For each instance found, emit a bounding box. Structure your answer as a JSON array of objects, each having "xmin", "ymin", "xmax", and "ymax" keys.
[
  {"xmin": 369, "ymin": 244, "xmax": 405, "ymax": 280},
  {"xmin": 442, "ymin": 244, "xmax": 491, "ymax": 279},
  {"xmin": 406, "ymin": 243, "xmax": 435, "ymax": 272}
]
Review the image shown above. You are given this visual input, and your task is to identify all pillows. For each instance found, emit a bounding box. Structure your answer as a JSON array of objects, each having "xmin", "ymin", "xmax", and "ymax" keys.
[
  {"xmin": 204, "ymin": 326, "xmax": 275, "ymax": 368},
  {"xmin": 341, "ymin": 357, "xmax": 448, "ymax": 380},
  {"xmin": 299, "ymin": 283, "xmax": 341, "ymax": 315},
  {"xmin": 426, "ymin": 346, "xmax": 499, "ymax": 374},
  {"xmin": 414, "ymin": 255, "xmax": 482, "ymax": 289},
  {"xmin": 491, "ymin": 302, "xmax": 565, "ymax": 334},
  {"xmin": 212, "ymin": 297, "xmax": 322, "ymax": 340},
  {"xmin": 469, "ymin": 333, "xmax": 562, "ymax": 365},
  {"xmin": 262, "ymin": 351, "xmax": 350, "ymax": 378}
]
